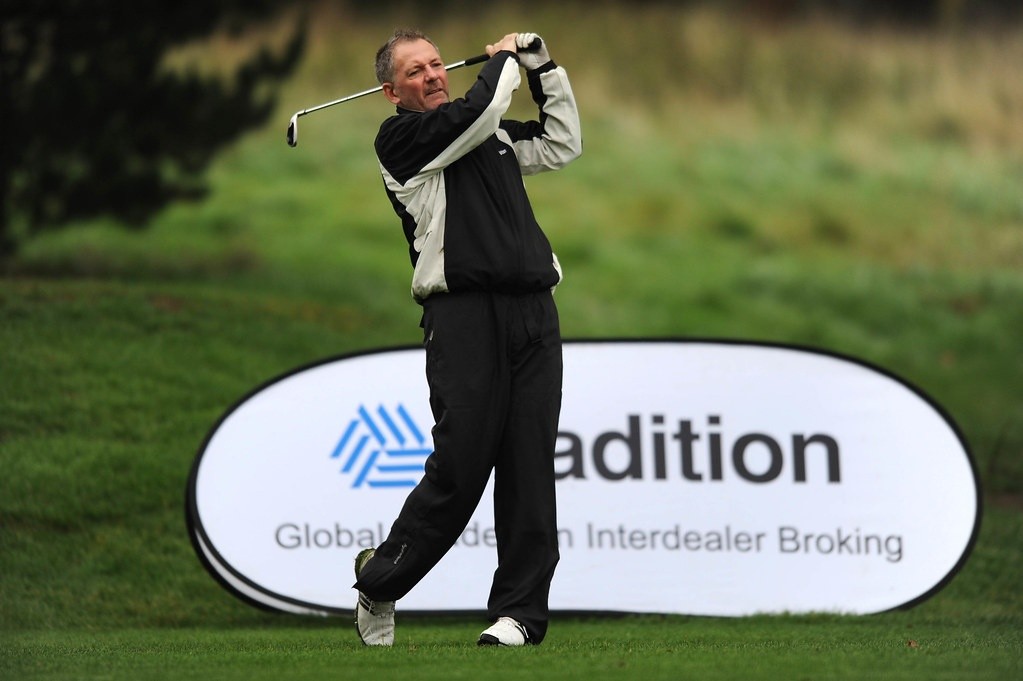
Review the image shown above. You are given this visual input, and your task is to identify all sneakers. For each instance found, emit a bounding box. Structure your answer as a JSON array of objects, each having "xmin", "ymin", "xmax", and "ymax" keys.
[
  {"xmin": 478, "ymin": 617, "xmax": 531, "ymax": 647},
  {"xmin": 352, "ymin": 548, "xmax": 395, "ymax": 649}
]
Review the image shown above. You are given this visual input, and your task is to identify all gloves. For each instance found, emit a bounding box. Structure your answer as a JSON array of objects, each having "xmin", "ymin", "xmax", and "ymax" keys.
[{"xmin": 515, "ymin": 32, "xmax": 551, "ymax": 70}]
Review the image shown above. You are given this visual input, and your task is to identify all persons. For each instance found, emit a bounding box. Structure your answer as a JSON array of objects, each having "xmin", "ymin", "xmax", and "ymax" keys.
[{"xmin": 352, "ymin": 30, "xmax": 586, "ymax": 647}]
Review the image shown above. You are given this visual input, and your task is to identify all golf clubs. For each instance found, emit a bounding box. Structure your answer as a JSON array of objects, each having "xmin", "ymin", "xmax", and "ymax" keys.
[{"xmin": 287, "ymin": 35, "xmax": 543, "ymax": 148}]
